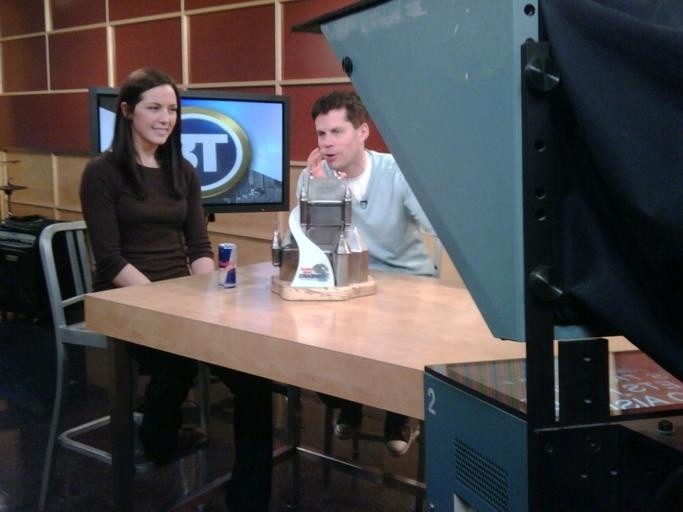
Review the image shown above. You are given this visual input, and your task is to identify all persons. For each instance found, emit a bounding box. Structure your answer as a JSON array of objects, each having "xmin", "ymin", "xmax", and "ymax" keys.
[
  {"xmin": 80, "ymin": 66, "xmax": 273, "ymax": 511},
  {"xmin": 296, "ymin": 92, "xmax": 438, "ymax": 456}
]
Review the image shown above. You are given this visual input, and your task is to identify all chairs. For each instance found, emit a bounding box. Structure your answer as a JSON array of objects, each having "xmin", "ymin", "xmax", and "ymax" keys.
[
  {"xmin": 38, "ymin": 220, "xmax": 212, "ymax": 511},
  {"xmin": 317, "ymin": 396, "xmax": 425, "ymax": 496}
]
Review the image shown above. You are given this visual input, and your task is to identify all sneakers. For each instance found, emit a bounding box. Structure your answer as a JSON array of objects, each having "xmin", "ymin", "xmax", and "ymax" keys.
[
  {"xmin": 334, "ymin": 405, "xmax": 363, "ymax": 440},
  {"xmin": 385, "ymin": 416, "xmax": 413, "ymax": 457}
]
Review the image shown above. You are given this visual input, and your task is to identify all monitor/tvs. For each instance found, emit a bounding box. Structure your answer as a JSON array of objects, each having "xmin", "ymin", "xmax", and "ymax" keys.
[{"xmin": 89, "ymin": 87, "xmax": 290, "ymax": 212}]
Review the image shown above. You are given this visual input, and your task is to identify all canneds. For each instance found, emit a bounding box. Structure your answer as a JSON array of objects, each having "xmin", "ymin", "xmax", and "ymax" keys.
[{"xmin": 217, "ymin": 243, "xmax": 237, "ymax": 288}]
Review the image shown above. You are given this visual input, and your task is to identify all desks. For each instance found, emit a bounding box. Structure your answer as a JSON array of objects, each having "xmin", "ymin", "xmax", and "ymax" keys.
[{"xmin": 84, "ymin": 263, "xmax": 638, "ymax": 511}]
